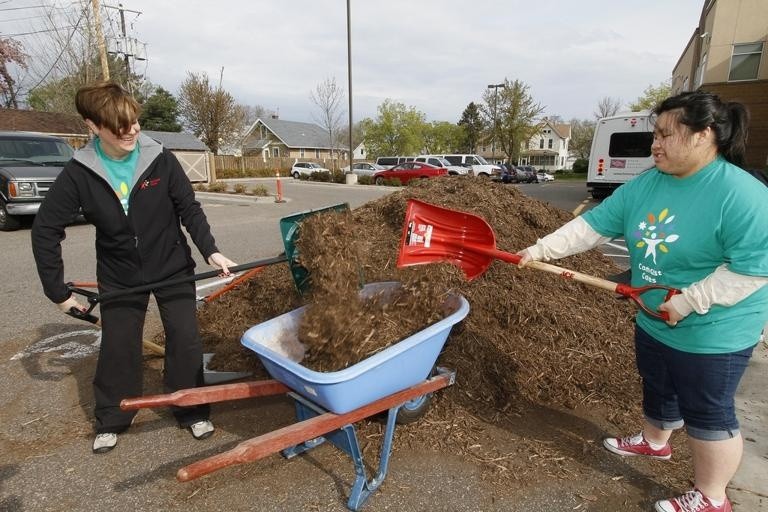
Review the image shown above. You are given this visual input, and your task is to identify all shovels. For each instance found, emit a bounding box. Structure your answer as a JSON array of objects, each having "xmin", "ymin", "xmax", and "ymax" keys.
[
  {"xmin": 67, "ymin": 202, "xmax": 365, "ymax": 319},
  {"xmin": 397, "ymin": 198, "xmax": 684, "ymax": 320},
  {"xmin": 604, "ymin": 268, "xmax": 632, "ymax": 300},
  {"xmin": 64, "ymin": 306, "xmax": 253, "ymax": 384}
]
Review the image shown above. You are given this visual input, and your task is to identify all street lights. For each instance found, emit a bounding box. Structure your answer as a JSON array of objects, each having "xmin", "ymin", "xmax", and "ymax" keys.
[{"xmin": 487, "ymin": 83, "xmax": 505, "ymax": 164}]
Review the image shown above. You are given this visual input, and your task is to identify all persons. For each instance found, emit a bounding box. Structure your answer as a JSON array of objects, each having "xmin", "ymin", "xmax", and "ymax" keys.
[
  {"xmin": 28, "ymin": 78, "xmax": 242, "ymax": 455},
  {"xmin": 512, "ymin": 90, "xmax": 768, "ymax": 512}
]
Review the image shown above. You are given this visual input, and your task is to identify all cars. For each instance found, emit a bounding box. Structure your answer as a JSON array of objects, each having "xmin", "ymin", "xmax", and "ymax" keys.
[
  {"xmin": 372, "ymin": 161, "xmax": 448, "ymax": 186},
  {"xmin": 339, "ymin": 162, "xmax": 386, "ymax": 177},
  {"xmin": 494, "ymin": 163, "xmax": 554, "ymax": 184}
]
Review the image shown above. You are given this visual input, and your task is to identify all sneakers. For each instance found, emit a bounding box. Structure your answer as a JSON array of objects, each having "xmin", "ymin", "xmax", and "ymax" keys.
[
  {"xmin": 192, "ymin": 419, "xmax": 214, "ymax": 440},
  {"xmin": 602, "ymin": 431, "xmax": 672, "ymax": 460},
  {"xmin": 655, "ymin": 487, "xmax": 732, "ymax": 512},
  {"xmin": 92, "ymin": 432, "xmax": 118, "ymax": 453}
]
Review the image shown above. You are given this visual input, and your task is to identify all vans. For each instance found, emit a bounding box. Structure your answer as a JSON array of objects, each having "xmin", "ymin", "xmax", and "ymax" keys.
[
  {"xmin": 374, "ymin": 156, "xmax": 469, "ymax": 180},
  {"xmin": 586, "ymin": 114, "xmax": 657, "ymax": 200},
  {"xmin": 418, "ymin": 153, "xmax": 502, "ymax": 182}
]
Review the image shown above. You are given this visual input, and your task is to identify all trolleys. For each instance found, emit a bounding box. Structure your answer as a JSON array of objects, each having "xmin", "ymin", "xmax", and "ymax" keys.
[{"xmin": 116, "ymin": 282, "xmax": 472, "ymax": 511}]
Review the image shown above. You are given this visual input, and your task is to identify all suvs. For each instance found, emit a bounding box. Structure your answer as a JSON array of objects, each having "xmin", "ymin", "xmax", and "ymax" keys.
[
  {"xmin": 0, "ymin": 130, "xmax": 82, "ymax": 230},
  {"xmin": 290, "ymin": 161, "xmax": 330, "ymax": 179}
]
